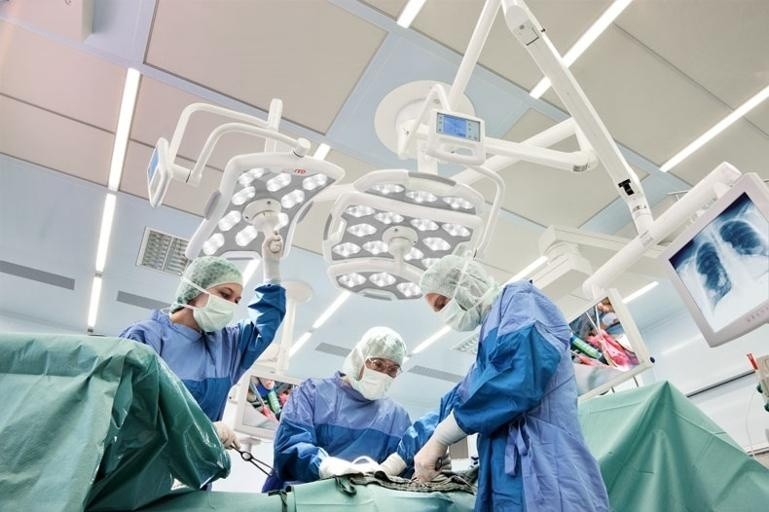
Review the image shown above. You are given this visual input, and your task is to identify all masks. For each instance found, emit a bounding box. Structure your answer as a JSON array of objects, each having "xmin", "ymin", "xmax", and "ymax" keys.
[
  {"xmin": 186, "ymin": 285, "xmax": 239, "ymax": 333},
  {"xmin": 436, "ymin": 287, "xmax": 485, "ymax": 332},
  {"xmin": 350, "ymin": 355, "xmax": 394, "ymax": 400}
]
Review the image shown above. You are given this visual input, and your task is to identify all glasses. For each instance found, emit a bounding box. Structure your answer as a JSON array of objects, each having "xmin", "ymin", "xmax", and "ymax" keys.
[{"xmin": 369, "ymin": 359, "xmax": 401, "ymax": 377}]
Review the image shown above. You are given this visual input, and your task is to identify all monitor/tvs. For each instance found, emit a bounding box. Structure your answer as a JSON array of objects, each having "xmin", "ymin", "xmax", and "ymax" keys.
[
  {"xmin": 657, "ymin": 172, "xmax": 769, "ymax": 347},
  {"xmin": 233, "ymin": 369, "xmax": 305, "ymax": 439},
  {"xmin": 566, "ymin": 288, "xmax": 653, "ymax": 402}
]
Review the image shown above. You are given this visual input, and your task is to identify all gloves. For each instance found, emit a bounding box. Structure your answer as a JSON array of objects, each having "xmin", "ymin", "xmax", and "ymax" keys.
[
  {"xmin": 414, "ymin": 412, "xmax": 467, "ymax": 482},
  {"xmin": 262, "ymin": 230, "xmax": 283, "ymax": 285},
  {"xmin": 318, "ymin": 457, "xmax": 362, "ymax": 478},
  {"xmin": 380, "ymin": 452, "xmax": 406, "ymax": 476},
  {"xmin": 213, "ymin": 421, "xmax": 241, "ymax": 450}
]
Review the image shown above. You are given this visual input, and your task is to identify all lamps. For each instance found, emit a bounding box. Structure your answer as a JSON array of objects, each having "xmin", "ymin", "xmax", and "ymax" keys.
[
  {"xmin": 185, "ymin": 151, "xmax": 345, "ymax": 262},
  {"xmin": 323, "ymin": 153, "xmax": 489, "ymax": 301}
]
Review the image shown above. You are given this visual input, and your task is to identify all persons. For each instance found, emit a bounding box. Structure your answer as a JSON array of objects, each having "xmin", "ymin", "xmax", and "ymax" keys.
[
  {"xmin": 247, "ymin": 375, "xmax": 275, "ymax": 403},
  {"xmin": 377, "ymin": 255, "xmax": 609, "ymax": 512},
  {"xmin": 117, "ymin": 227, "xmax": 286, "ymax": 449},
  {"xmin": 597, "ymin": 298, "xmax": 615, "ymax": 313},
  {"xmin": 262, "ymin": 325, "xmax": 412, "ymax": 492}
]
